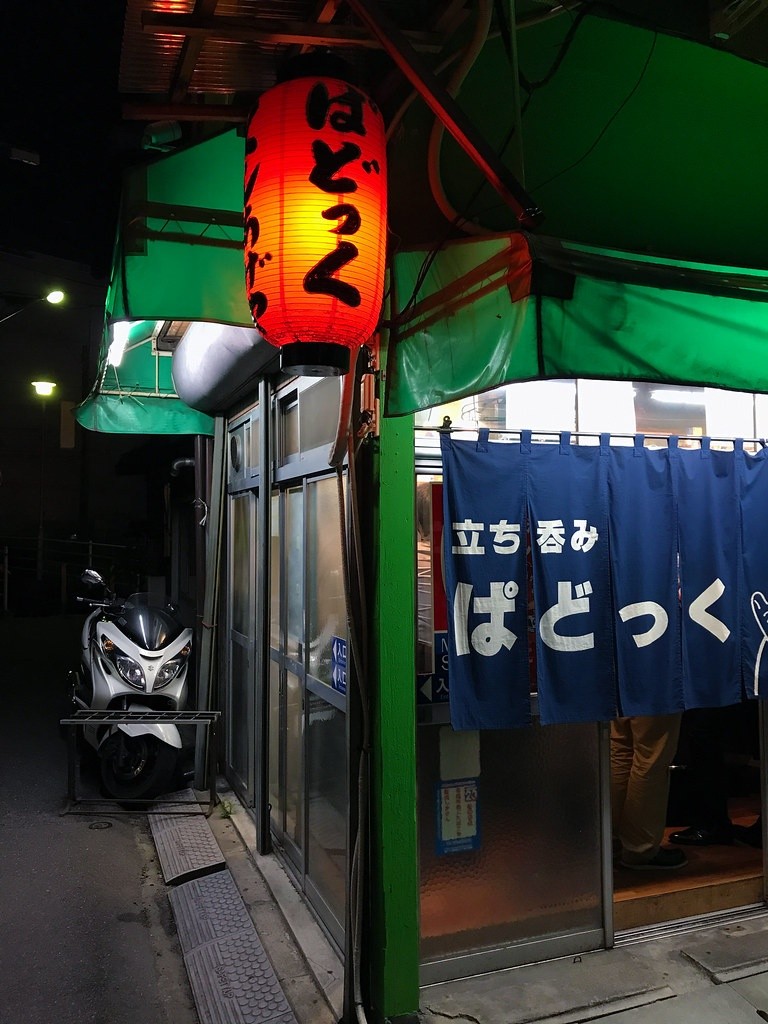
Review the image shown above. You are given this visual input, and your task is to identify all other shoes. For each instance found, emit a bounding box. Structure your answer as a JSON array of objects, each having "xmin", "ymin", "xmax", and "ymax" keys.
[{"xmin": 611, "ymin": 836, "xmax": 687, "ymax": 870}]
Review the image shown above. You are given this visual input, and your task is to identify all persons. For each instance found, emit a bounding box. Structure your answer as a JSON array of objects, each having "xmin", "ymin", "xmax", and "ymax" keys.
[
  {"xmin": 413, "ymin": 478, "xmax": 436, "ymax": 675},
  {"xmin": 608, "ymin": 713, "xmax": 691, "ymax": 872}
]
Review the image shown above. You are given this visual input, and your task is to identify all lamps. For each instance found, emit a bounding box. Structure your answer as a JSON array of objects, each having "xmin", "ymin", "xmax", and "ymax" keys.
[
  {"xmin": 31, "ymin": 382, "xmax": 56, "ymax": 395},
  {"xmin": 46, "ymin": 290, "xmax": 64, "ymax": 304}
]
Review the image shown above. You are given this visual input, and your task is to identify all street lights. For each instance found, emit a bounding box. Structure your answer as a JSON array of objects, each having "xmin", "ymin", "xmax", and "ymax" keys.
[{"xmin": 31, "ymin": 378, "xmax": 58, "ymax": 585}]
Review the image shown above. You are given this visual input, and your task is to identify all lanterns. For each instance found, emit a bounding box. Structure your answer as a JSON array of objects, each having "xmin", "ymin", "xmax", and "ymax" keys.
[{"xmin": 243, "ymin": 75, "xmax": 390, "ymax": 376}]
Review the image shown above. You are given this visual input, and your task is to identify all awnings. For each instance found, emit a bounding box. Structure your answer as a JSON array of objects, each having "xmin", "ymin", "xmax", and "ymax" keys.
[{"xmin": 70, "ymin": 120, "xmax": 240, "ymax": 436}]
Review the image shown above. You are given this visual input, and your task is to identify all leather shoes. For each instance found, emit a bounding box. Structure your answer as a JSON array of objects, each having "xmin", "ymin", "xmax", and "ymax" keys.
[
  {"xmin": 732, "ymin": 813, "xmax": 762, "ymax": 849},
  {"xmin": 669, "ymin": 819, "xmax": 735, "ymax": 846}
]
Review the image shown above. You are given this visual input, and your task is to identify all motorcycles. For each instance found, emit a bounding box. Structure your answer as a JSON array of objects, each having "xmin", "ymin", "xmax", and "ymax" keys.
[{"xmin": 65, "ymin": 568, "xmax": 198, "ymax": 807}]
